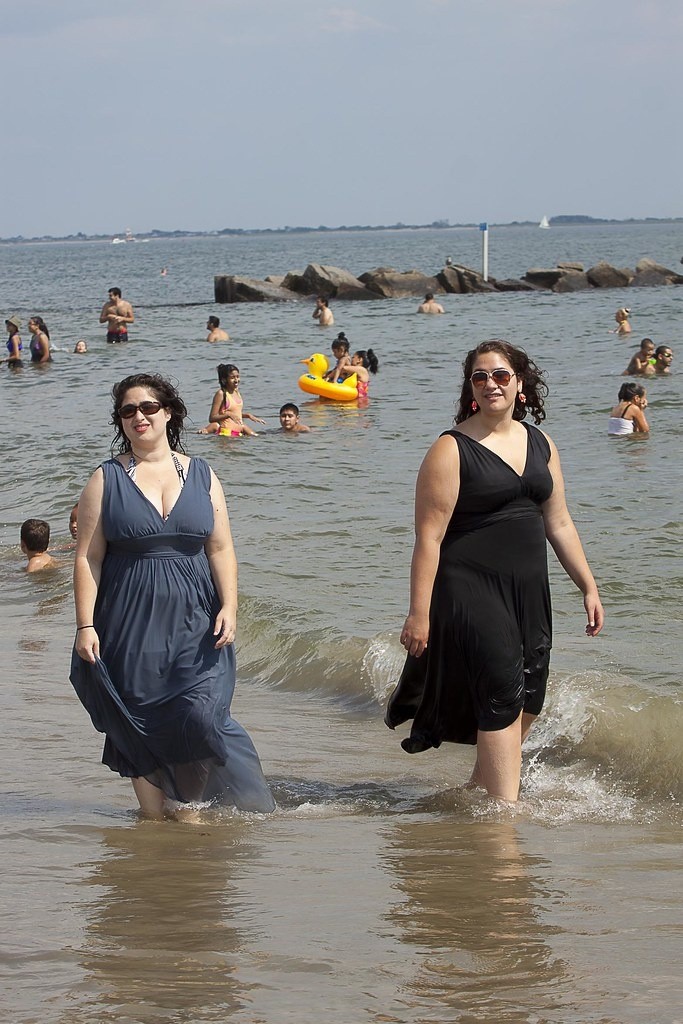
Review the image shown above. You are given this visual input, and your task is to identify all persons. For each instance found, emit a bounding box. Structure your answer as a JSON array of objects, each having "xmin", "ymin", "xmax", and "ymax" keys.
[
  {"xmin": 75, "ymin": 340, "xmax": 87, "ymax": 353},
  {"xmin": 207, "ymin": 316, "xmax": 229, "ymax": 343},
  {"xmin": 21, "ymin": 519, "xmax": 52, "ymax": 573},
  {"xmin": 280, "ymin": 403, "xmax": 309, "ymax": 431},
  {"xmin": 609, "ymin": 308, "xmax": 631, "ymax": 333},
  {"xmin": 68, "ymin": 373, "xmax": 275, "ymax": 821},
  {"xmin": 313, "ymin": 296, "xmax": 333, "ymax": 325},
  {"xmin": 418, "ymin": 294, "xmax": 444, "ymax": 314},
  {"xmin": 198, "ymin": 364, "xmax": 266, "ymax": 437},
  {"xmin": 160, "ymin": 269, "xmax": 166, "ymax": 275},
  {"xmin": 324, "ymin": 331, "xmax": 379, "ymax": 398},
  {"xmin": 28, "ymin": 316, "xmax": 54, "ymax": 362},
  {"xmin": 608, "ymin": 382, "xmax": 650, "ymax": 435},
  {"xmin": 0, "ymin": 316, "xmax": 23, "ymax": 369},
  {"xmin": 624, "ymin": 338, "xmax": 673, "ymax": 375},
  {"xmin": 69, "ymin": 503, "xmax": 79, "ymax": 540},
  {"xmin": 383, "ymin": 340, "xmax": 604, "ymax": 802},
  {"xmin": 99, "ymin": 287, "xmax": 134, "ymax": 343}
]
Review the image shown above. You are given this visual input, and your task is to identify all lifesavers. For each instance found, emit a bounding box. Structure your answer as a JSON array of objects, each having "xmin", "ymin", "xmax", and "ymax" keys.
[{"xmin": 298, "ymin": 353, "xmax": 359, "ymax": 401}]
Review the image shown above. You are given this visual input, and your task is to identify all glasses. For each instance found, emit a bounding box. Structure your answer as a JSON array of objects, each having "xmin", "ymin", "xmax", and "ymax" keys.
[
  {"xmin": 468, "ymin": 368, "xmax": 515, "ymax": 386},
  {"xmin": 118, "ymin": 399, "xmax": 165, "ymax": 417},
  {"xmin": 27, "ymin": 322, "xmax": 36, "ymax": 326},
  {"xmin": 662, "ymin": 353, "xmax": 673, "ymax": 357}
]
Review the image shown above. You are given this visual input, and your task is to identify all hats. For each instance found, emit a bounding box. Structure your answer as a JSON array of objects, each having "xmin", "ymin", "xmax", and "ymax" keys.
[{"xmin": 5, "ymin": 316, "xmax": 21, "ymax": 331}]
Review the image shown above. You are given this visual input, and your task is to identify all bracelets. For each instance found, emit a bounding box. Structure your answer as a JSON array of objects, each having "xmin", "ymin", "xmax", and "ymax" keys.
[
  {"xmin": 77, "ymin": 626, "xmax": 94, "ymax": 630},
  {"xmin": 4, "ymin": 358, "xmax": 6, "ymax": 362}
]
